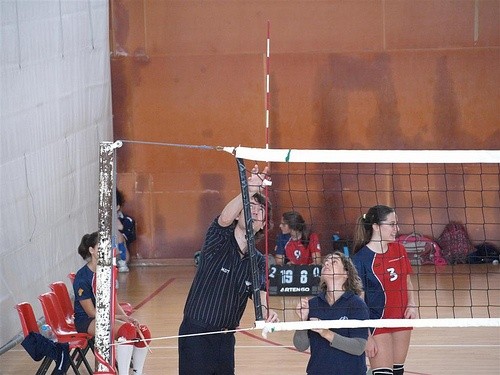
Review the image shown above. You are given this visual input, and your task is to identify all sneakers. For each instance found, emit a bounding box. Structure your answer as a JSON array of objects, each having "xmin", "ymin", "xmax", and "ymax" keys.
[{"xmin": 118, "ymin": 260, "xmax": 128, "ymax": 273}]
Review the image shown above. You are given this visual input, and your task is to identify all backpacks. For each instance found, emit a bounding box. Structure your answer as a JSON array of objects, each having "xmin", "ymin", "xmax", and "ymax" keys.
[{"xmin": 118, "ymin": 210, "xmax": 136, "ymax": 243}]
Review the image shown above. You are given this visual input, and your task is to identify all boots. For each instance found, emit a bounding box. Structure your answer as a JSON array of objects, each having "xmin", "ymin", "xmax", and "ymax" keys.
[{"xmin": 115, "ymin": 344, "xmax": 148, "ymax": 375}]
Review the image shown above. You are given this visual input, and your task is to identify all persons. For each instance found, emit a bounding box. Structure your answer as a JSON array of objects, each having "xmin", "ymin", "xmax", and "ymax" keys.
[
  {"xmin": 349, "ymin": 206, "xmax": 416, "ymax": 375},
  {"xmin": 178, "ymin": 164, "xmax": 280, "ymax": 375},
  {"xmin": 295, "ymin": 252, "xmax": 370, "ymax": 375},
  {"xmin": 74, "ymin": 231, "xmax": 151, "ymax": 375},
  {"xmin": 274, "ymin": 212, "xmax": 321, "ymax": 265},
  {"xmin": 114, "ymin": 187, "xmax": 136, "ymax": 272}
]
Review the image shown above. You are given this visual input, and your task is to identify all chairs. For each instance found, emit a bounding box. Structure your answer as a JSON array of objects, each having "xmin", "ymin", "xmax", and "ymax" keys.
[{"xmin": 15, "ymin": 273, "xmax": 133, "ymax": 375}]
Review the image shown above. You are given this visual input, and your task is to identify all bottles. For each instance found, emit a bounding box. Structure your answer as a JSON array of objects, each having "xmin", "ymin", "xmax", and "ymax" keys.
[{"xmin": 40, "ymin": 324, "xmax": 58, "ymax": 342}]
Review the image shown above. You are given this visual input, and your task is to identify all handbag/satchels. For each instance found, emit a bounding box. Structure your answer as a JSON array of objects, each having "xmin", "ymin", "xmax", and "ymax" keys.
[{"xmin": 396, "ymin": 222, "xmax": 500, "ymax": 266}]
